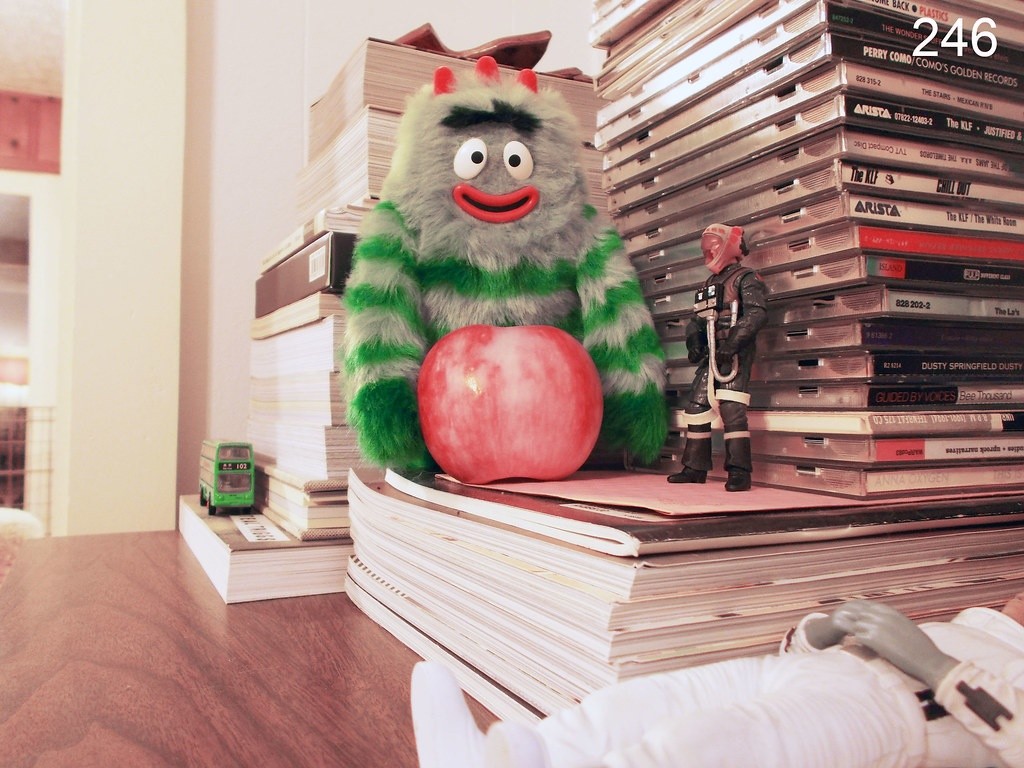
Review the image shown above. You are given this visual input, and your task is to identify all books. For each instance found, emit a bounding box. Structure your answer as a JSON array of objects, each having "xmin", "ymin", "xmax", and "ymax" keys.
[
  {"xmin": 345, "ymin": 467, "xmax": 1024, "ymax": 725},
  {"xmin": 179, "ymin": 37, "xmax": 610, "ymax": 605}
]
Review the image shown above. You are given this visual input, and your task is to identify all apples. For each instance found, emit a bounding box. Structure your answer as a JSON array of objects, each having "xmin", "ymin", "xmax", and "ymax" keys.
[{"xmin": 417, "ymin": 327, "xmax": 600, "ymax": 482}]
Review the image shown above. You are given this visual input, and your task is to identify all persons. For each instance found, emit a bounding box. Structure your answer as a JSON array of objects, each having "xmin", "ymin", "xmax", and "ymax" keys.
[
  {"xmin": 667, "ymin": 224, "xmax": 768, "ymax": 491},
  {"xmin": 411, "ymin": 591, "xmax": 1024, "ymax": 768}
]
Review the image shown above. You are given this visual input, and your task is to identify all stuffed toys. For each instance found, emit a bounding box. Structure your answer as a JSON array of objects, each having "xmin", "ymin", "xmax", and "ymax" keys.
[{"xmin": 342, "ymin": 56, "xmax": 667, "ymax": 474}]
